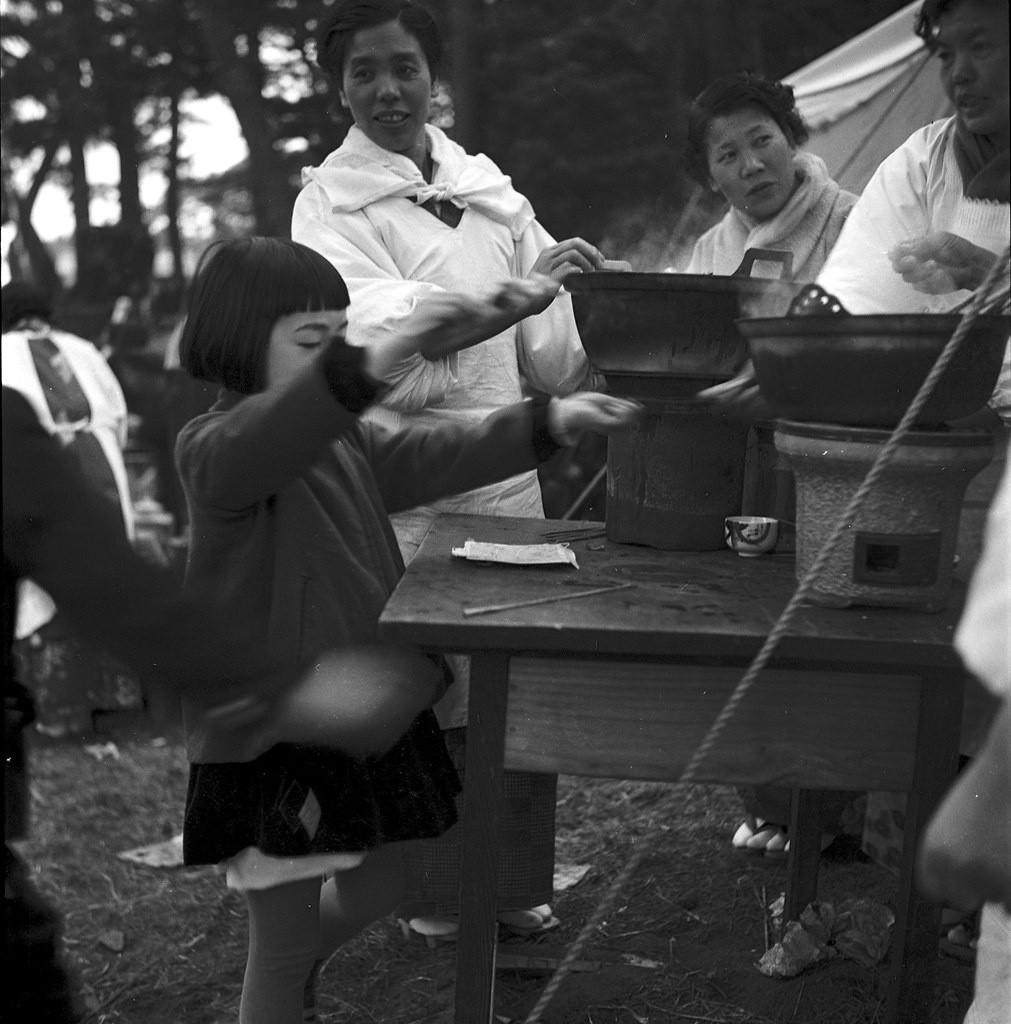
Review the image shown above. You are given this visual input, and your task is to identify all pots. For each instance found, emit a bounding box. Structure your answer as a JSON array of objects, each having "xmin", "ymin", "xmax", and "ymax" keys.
[
  {"xmin": 732, "ymin": 283, "xmax": 1011, "ymax": 429},
  {"xmin": 560, "ymin": 248, "xmax": 808, "ymax": 377}
]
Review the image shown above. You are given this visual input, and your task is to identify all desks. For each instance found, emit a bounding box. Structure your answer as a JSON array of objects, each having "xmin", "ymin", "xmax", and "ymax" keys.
[{"xmin": 376, "ymin": 514, "xmax": 972, "ymax": 1024}]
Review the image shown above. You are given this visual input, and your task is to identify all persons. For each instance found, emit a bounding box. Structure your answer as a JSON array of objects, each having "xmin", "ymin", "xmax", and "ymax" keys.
[
  {"xmin": 174, "ymin": 236, "xmax": 644, "ymax": 1023},
  {"xmin": 0, "ymin": 281, "xmax": 181, "ymax": 1024},
  {"xmin": 289, "ymin": 0, "xmax": 635, "ymax": 939},
  {"xmin": 684, "ymin": 1, "xmax": 1011, "ymax": 1024}
]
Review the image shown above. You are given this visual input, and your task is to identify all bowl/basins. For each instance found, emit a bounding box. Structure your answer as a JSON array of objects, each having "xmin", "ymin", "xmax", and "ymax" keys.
[{"xmin": 725, "ymin": 516, "xmax": 779, "ymax": 557}]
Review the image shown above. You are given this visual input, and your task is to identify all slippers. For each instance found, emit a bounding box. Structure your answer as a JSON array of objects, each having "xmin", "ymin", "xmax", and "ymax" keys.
[
  {"xmin": 764, "ymin": 824, "xmax": 841, "ymax": 861},
  {"xmin": 731, "ymin": 808, "xmax": 788, "ymax": 854},
  {"xmin": 496, "ymin": 902, "xmax": 562, "ymax": 942}
]
